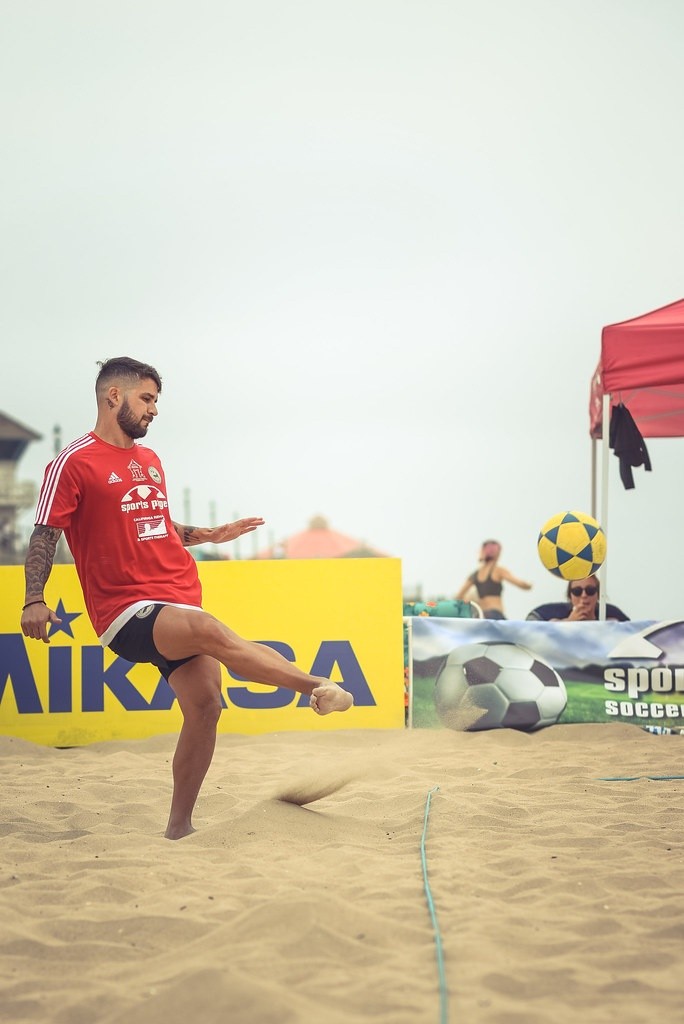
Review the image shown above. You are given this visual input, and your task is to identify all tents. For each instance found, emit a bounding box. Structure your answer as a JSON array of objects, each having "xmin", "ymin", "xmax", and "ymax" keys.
[{"xmin": 590, "ymin": 299, "xmax": 684, "ymax": 620}]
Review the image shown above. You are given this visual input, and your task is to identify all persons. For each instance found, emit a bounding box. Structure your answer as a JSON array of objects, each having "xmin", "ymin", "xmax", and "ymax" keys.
[
  {"xmin": 20, "ymin": 357, "xmax": 354, "ymax": 839},
  {"xmin": 551, "ymin": 574, "xmax": 616, "ymax": 622},
  {"xmin": 456, "ymin": 540, "xmax": 532, "ymax": 621}
]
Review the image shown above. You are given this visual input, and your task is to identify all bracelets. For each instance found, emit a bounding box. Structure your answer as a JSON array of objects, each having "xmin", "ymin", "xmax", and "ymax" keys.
[{"xmin": 22, "ymin": 601, "xmax": 46, "ymax": 610}]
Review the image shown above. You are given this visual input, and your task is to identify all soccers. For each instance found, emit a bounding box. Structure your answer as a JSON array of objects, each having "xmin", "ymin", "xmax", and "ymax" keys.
[
  {"xmin": 433, "ymin": 640, "xmax": 567, "ymax": 733},
  {"xmin": 537, "ymin": 510, "xmax": 609, "ymax": 583}
]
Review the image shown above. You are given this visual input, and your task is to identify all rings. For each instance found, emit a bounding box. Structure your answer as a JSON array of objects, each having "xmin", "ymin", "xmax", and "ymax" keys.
[{"xmin": 572, "ymin": 606, "xmax": 578, "ymax": 612}]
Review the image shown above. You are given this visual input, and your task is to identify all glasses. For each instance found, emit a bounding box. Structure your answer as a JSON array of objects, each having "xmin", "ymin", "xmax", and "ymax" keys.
[{"xmin": 570, "ymin": 586, "xmax": 598, "ymax": 596}]
